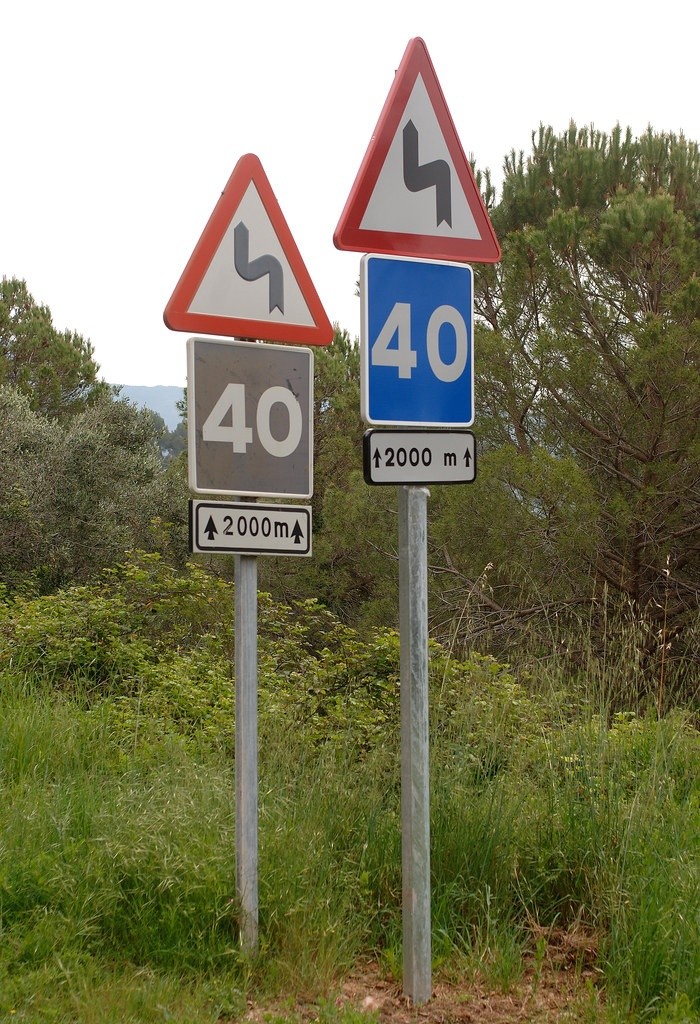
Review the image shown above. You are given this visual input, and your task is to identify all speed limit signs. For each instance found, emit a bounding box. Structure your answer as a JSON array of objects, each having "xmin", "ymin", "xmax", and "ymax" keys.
[
  {"xmin": 359, "ymin": 250, "xmax": 478, "ymax": 428},
  {"xmin": 186, "ymin": 337, "xmax": 314, "ymax": 500}
]
